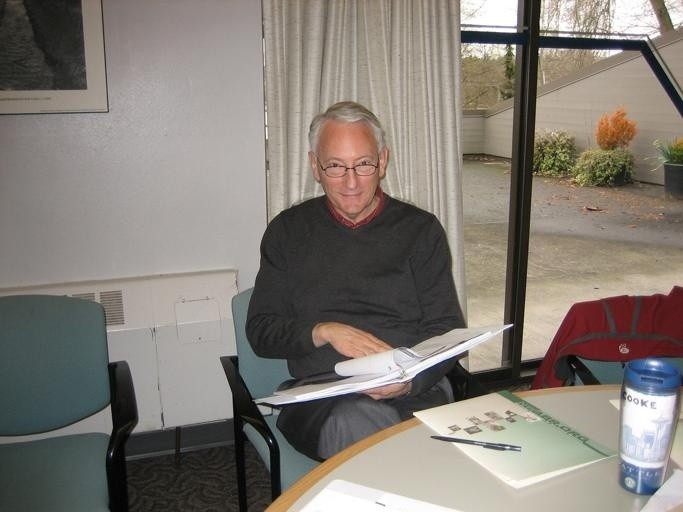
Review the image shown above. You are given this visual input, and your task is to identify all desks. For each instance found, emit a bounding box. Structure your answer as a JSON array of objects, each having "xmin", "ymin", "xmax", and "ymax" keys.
[{"xmin": 263, "ymin": 384, "xmax": 683, "ymax": 512}]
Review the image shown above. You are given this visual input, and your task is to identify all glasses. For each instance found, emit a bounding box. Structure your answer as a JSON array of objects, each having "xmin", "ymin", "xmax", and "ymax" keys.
[{"xmin": 317, "ymin": 156, "xmax": 380, "ymax": 178}]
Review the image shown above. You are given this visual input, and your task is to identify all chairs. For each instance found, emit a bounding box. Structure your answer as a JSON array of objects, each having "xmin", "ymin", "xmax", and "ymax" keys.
[
  {"xmin": 1, "ymin": 296, "xmax": 138, "ymax": 512},
  {"xmin": 219, "ymin": 286, "xmax": 324, "ymax": 512},
  {"xmin": 554, "ymin": 296, "xmax": 683, "ymax": 387}
]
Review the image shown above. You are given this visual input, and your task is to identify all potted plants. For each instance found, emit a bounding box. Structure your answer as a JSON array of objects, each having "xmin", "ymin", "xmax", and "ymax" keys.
[
  {"xmin": 642, "ymin": 138, "xmax": 683, "ymax": 204},
  {"xmin": 573, "ymin": 104, "xmax": 639, "ymax": 188}
]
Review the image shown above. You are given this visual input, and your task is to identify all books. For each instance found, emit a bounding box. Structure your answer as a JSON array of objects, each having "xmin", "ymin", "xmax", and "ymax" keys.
[
  {"xmin": 414, "ymin": 388, "xmax": 619, "ymax": 489},
  {"xmin": 251, "ymin": 324, "xmax": 514, "ymax": 407}
]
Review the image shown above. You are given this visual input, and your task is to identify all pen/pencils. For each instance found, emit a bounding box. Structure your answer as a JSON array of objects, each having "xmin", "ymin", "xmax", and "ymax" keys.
[{"xmin": 431, "ymin": 436, "xmax": 521, "ymax": 451}]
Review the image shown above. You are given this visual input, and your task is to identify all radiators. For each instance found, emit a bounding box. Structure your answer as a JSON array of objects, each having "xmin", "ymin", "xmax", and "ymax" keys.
[{"xmin": 1, "ymin": 267, "xmax": 240, "ymax": 467}]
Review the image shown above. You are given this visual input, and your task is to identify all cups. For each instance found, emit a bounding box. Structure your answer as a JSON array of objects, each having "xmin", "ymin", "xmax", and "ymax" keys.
[{"xmin": 618, "ymin": 356, "xmax": 683, "ymax": 497}]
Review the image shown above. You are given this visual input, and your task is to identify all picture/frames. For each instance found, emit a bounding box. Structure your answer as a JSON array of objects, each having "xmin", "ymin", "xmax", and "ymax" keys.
[{"xmin": 0, "ymin": 0, "xmax": 110, "ymax": 115}]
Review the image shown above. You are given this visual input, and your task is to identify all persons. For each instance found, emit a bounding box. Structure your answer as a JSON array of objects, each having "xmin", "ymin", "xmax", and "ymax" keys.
[{"xmin": 244, "ymin": 101, "xmax": 467, "ymax": 462}]
